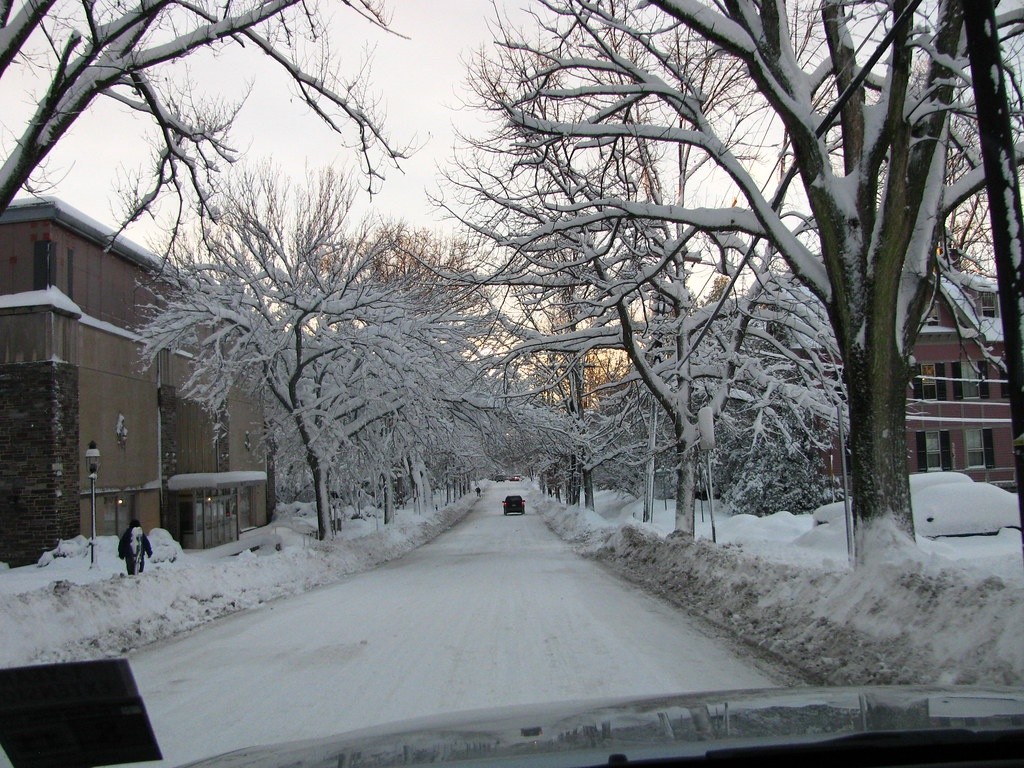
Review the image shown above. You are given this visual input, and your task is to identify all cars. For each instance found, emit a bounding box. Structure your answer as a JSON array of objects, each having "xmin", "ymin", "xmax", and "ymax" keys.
[
  {"xmin": 510, "ymin": 476, "xmax": 520, "ymax": 481},
  {"xmin": 502, "ymin": 495, "xmax": 525, "ymax": 516},
  {"xmin": 496, "ymin": 475, "xmax": 504, "ymax": 482},
  {"xmin": 812, "ymin": 472, "xmax": 1020, "ymax": 540}
]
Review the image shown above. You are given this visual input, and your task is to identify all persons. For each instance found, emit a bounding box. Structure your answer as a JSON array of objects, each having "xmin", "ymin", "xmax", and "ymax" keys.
[
  {"xmin": 476, "ymin": 487, "xmax": 481, "ymax": 497},
  {"xmin": 118, "ymin": 518, "xmax": 153, "ymax": 575}
]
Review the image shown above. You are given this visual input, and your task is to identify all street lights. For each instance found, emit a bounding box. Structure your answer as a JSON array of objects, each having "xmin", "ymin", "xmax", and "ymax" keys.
[
  {"xmin": 86, "ymin": 440, "xmax": 101, "ymax": 572},
  {"xmin": 698, "ymin": 407, "xmax": 717, "ymax": 543}
]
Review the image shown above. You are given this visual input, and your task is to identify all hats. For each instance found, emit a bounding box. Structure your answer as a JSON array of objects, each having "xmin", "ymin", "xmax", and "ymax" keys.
[{"xmin": 128, "ymin": 519, "xmax": 141, "ymax": 527}]
